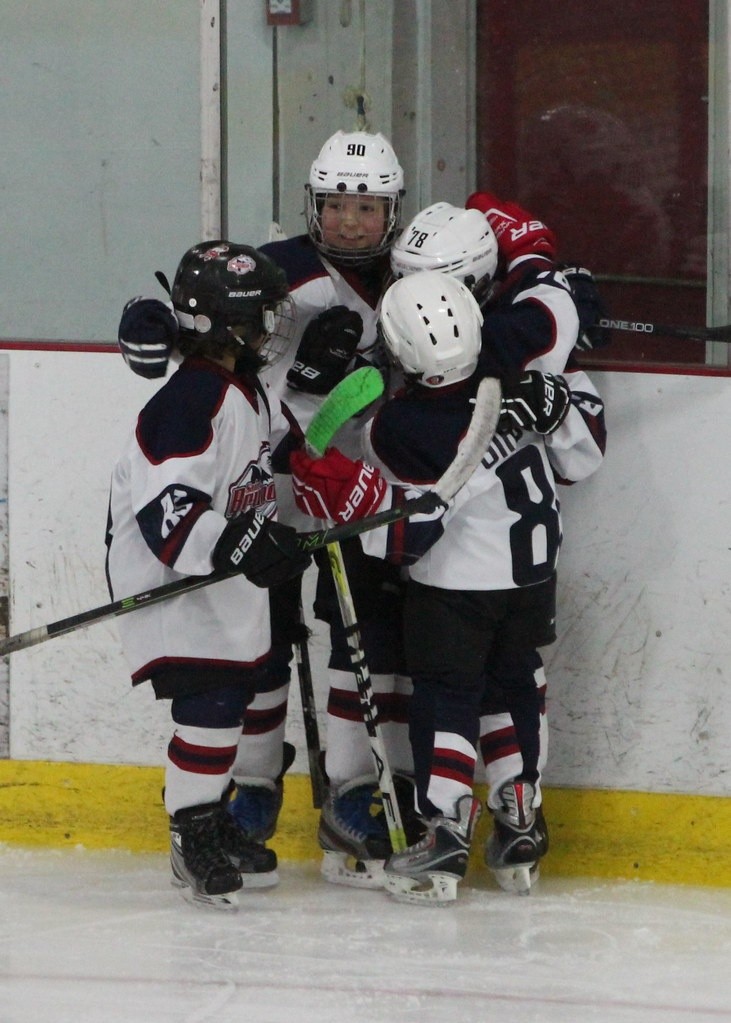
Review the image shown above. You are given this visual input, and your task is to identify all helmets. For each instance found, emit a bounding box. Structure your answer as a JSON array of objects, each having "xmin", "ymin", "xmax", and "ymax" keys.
[
  {"xmin": 172, "ymin": 239, "xmax": 299, "ymax": 371},
  {"xmin": 391, "ymin": 200, "xmax": 499, "ymax": 294},
  {"xmin": 302, "ymin": 129, "xmax": 405, "ymax": 264},
  {"xmin": 377, "ymin": 271, "xmax": 485, "ymax": 389}
]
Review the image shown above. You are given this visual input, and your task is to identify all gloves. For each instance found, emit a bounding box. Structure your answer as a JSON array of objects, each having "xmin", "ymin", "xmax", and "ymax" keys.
[
  {"xmin": 118, "ymin": 295, "xmax": 177, "ymax": 380},
  {"xmin": 212, "ymin": 510, "xmax": 313, "ymax": 587},
  {"xmin": 466, "ymin": 193, "xmax": 559, "ymax": 262},
  {"xmin": 291, "ymin": 446, "xmax": 386, "ymax": 529},
  {"xmin": 553, "ymin": 262, "xmax": 612, "ymax": 352},
  {"xmin": 289, "ymin": 306, "xmax": 363, "ymax": 389},
  {"xmin": 493, "ymin": 370, "xmax": 571, "ymax": 436}
]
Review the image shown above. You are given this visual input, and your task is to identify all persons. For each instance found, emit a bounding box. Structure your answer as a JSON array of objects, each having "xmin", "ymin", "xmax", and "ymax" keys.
[{"xmin": 99, "ymin": 122, "xmax": 608, "ymax": 901}]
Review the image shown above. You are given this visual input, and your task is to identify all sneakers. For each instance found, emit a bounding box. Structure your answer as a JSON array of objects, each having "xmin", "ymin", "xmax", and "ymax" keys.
[
  {"xmin": 162, "ymin": 786, "xmax": 281, "ymax": 912},
  {"xmin": 230, "ymin": 740, "xmax": 296, "ymax": 843},
  {"xmin": 317, "ymin": 750, "xmax": 393, "ymax": 888},
  {"xmin": 486, "ymin": 781, "xmax": 543, "ymax": 900},
  {"xmin": 384, "ymin": 795, "xmax": 485, "ymax": 907}
]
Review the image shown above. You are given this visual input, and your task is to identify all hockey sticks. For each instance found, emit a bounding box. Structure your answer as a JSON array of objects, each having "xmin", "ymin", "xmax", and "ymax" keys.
[
  {"xmin": 303, "ymin": 364, "xmax": 409, "ymax": 856},
  {"xmin": 289, "ymin": 613, "xmax": 340, "ymax": 812},
  {"xmin": 1, "ymin": 377, "xmax": 502, "ymax": 663}
]
